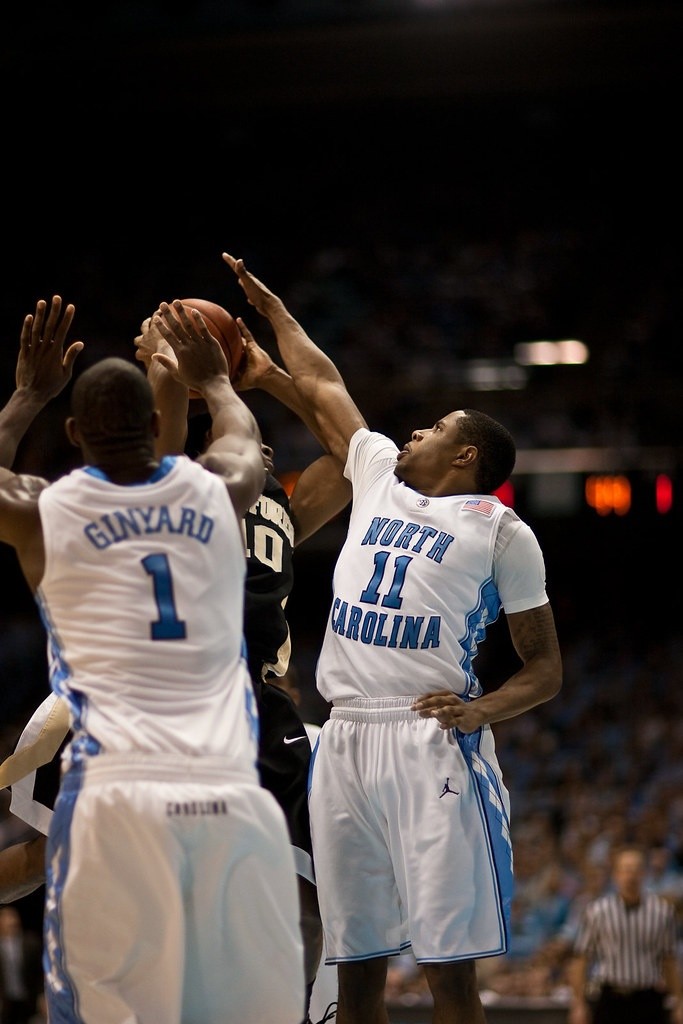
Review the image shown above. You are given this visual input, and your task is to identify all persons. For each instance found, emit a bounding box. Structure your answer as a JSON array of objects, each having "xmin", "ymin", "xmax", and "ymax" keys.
[
  {"xmin": 354, "ymin": 727, "xmax": 678, "ymax": 1007},
  {"xmin": 0, "ymin": 294, "xmax": 353, "ymax": 1024},
  {"xmin": 222, "ymin": 252, "xmax": 563, "ymax": 1024},
  {"xmin": 570, "ymin": 850, "xmax": 683, "ymax": 1024}
]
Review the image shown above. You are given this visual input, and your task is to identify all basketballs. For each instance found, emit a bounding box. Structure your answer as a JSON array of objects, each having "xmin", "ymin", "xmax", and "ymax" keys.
[{"xmin": 142, "ymin": 297, "xmax": 245, "ymax": 401}]
